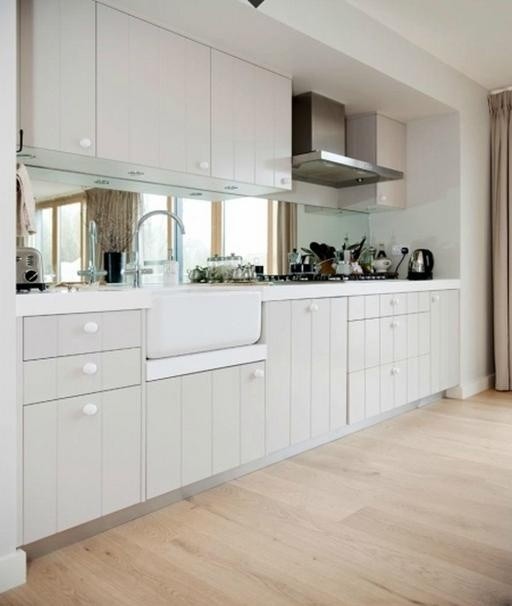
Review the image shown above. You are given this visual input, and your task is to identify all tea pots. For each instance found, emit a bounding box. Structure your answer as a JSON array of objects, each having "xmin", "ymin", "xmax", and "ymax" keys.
[{"xmin": 187, "ymin": 254, "xmax": 256, "ymax": 283}]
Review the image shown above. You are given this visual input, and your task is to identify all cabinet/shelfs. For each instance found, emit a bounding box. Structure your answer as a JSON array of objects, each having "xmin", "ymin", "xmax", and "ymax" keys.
[
  {"xmin": 261, "ymin": 296, "xmax": 347, "ymax": 468},
  {"xmin": 23, "ymin": 165, "xmax": 249, "ymax": 202},
  {"xmin": 21, "ymin": 310, "xmax": 143, "ymax": 562},
  {"xmin": 145, "ymin": 358, "xmax": 266, "ymax": 514},
  {"xmin": 210, "ymin": 46, "xmax": 293, "ymax": 198},
  {"xmin": 16, "ymin": 0, "xmax": 96, "ymax": 173},
  {"xmin": 432, "ymin": 288, "xmax": 461, "ymax": 401},
  {"xmin": 341, "ymin": 112, "xmax": 407, "ymax": 213},
  {"xmin": 347, "ymin": 292, "xmax": 433, "ymax": 433},
  {"xmin": 97, "ymin": 5, "xmax": 212, "ymax": 178}
]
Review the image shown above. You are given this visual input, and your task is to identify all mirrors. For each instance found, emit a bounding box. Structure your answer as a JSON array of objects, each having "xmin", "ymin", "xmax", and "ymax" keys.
[{"xmin": 16, "ymin": 164, "xmax": 369, "ymax": 284}]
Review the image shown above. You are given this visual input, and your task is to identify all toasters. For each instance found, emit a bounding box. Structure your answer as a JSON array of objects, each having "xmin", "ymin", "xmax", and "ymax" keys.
[{"xmin": 16, "ymin": 248, "xmax": 45, "ymax": 291}]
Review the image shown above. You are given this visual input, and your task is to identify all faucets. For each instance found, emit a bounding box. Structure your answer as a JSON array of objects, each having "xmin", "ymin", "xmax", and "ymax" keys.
[{"xmin": 136, "ymin": 209, "xmax": 185, "ymax": 270}]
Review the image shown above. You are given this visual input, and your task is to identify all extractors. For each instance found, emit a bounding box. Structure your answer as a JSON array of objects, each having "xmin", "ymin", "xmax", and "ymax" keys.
[{"xmin": 292, "ymin": 92, "xmax": 403, "ymax": 188}]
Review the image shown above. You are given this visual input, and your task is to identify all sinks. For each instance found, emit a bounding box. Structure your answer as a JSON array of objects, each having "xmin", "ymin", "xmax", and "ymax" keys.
[{"xmin": 140, "ymin": 282, "xmax": 261, "ymax": 292}]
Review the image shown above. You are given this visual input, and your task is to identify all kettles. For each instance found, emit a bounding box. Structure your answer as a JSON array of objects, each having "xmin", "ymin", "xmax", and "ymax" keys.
[{"xmin": 407, "ymin": 249, "xmax": 433, "ymax": 280}]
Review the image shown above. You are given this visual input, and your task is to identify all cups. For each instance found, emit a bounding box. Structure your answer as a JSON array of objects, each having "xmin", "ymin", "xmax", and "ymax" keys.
[{"xmin": 374, "ymin": 257, "xmax": 391, "ymax": 273}]
